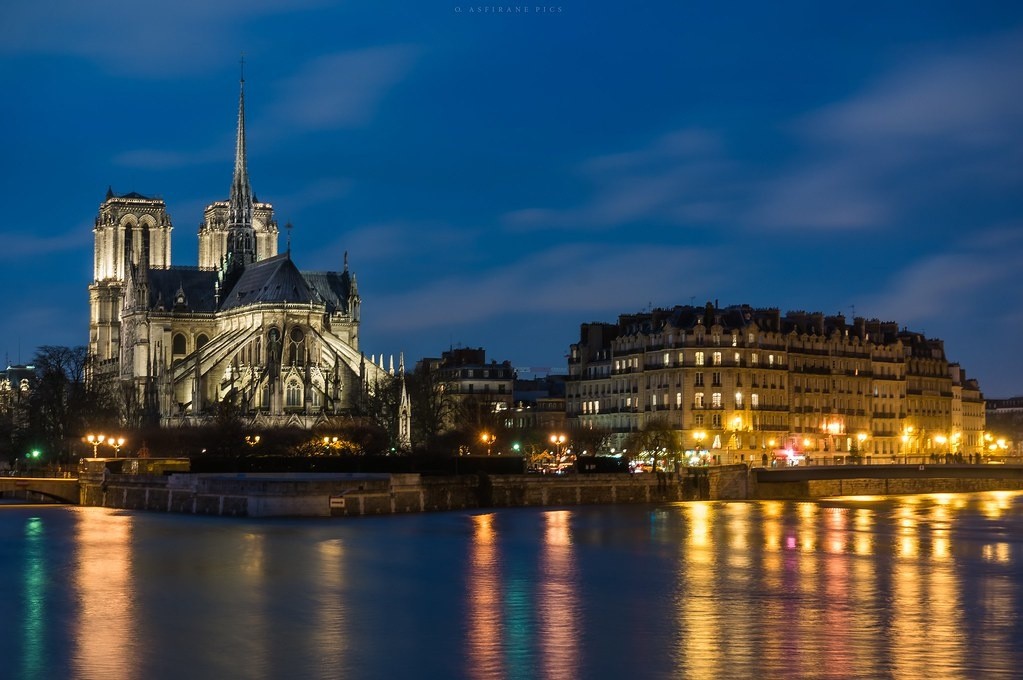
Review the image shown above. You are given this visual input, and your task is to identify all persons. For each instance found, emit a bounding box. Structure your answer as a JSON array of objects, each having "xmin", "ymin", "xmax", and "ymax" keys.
[{"xmin": 100, "ymin": 466, "xmax": 109, "ymax": 486}]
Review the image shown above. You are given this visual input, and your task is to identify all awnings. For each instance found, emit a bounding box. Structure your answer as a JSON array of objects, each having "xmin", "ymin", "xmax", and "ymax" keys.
[{"xmin": 772, "ymin": 450, "xmax": 803, "ymax": 457}]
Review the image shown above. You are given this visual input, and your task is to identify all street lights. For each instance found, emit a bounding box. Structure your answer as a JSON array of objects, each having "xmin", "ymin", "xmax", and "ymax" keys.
[
  {"xmin": 87, "ymin": 434, "xmax": 105, "ymax": 457},
  {"xmin": 551, "ymin": 436, "xmax": 566, "ymax": 467},
  {"xmin": 693, "ymin": 432, "xmax": 706, "ymax": 465},
  {"xmin": 482, "ymin": 434, "xmax": 497, "ymax": 456},
  {"xmin": 246, "ymin": 435, "xmax": 260, "ymax": 449},
  {"xmin": 108, "ymin": 437, "xmax": 125, "ymax": 457},
  {"xmin": 324, "ymin": 436, "xmax": 337, "ymax": 456}
]
